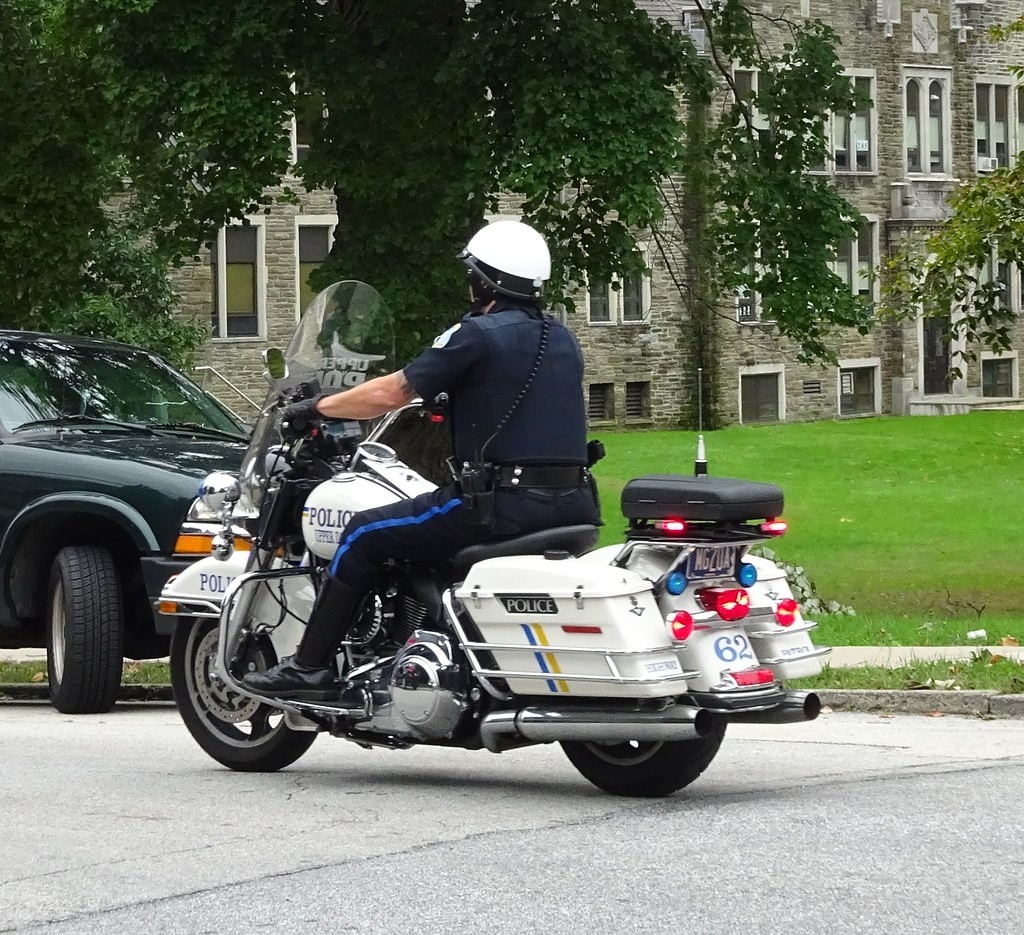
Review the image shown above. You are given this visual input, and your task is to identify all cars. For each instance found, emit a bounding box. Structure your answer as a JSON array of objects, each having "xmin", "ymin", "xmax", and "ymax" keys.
[{"xmin": 1, "ymin": 330, "xmax": 345, "ymax": 714}]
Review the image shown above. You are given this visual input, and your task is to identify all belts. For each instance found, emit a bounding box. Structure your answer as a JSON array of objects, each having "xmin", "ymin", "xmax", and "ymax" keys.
[{"xmin": 496, "ymin": 468, "xmax": 581, "ymax": 487}]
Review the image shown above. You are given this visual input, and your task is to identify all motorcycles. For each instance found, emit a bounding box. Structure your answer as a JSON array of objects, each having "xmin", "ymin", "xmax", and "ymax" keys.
[{"xmin": 150, "ymin": 279, "xmax": 829, "ymax": 798}]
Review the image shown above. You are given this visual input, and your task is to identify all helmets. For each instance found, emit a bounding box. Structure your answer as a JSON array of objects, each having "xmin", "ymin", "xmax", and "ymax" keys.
[{"xmin": 455, "ymin": 219, "xmax": 551, "ymax": 301}]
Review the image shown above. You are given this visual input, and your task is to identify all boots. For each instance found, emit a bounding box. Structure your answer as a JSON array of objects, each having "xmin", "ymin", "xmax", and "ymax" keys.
[{"xmin": 241, "ymin": 566, "xmax": 364, "ymax": 701}]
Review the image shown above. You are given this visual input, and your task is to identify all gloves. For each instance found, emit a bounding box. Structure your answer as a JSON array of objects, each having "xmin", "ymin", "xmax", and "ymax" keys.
[{"xmin": 280, "ymin": 394, "xmax": 335, "ymax": 447}]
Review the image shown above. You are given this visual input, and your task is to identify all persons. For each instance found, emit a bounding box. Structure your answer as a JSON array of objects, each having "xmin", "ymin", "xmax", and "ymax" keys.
[{"xmin": 242, "ymin": 220, "xmax": 607, "ymax": 701}]
